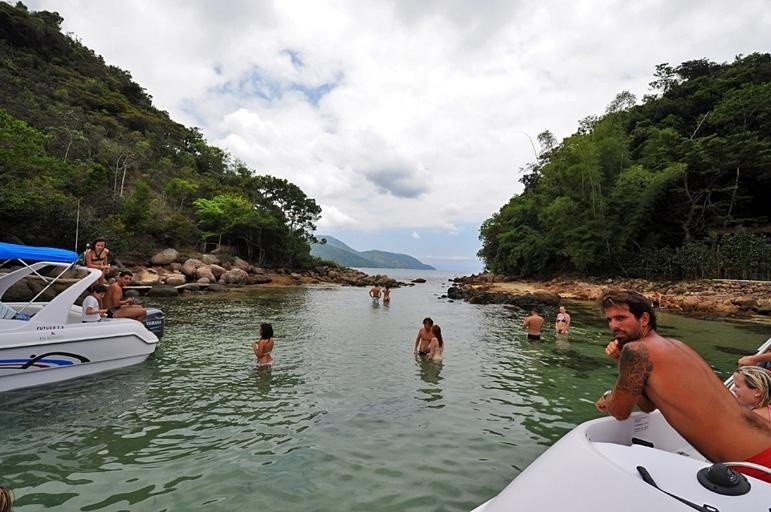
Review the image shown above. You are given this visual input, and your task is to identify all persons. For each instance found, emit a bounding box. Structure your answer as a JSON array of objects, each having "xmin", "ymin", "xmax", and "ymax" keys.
[
  {"xmin": 105, "ymin": 270, "xmax": 148, "ymax": 323},
  {"xmin": 427, "ymin": 324, "xmax": 445, "ymax": 361},
  {"xmin": 369, "ymin": 284, "xmax": 383, "ymax": 303},
  {"xmin": 253, "ymin": 323, "xmax": 275, "ymax": 365},
  {"xmin": 556, "ymin": 305, "xmax": 572, "ymax": 332},
  {"xmin": 413, "ymin": 318, "xmax": 435, "ymax": 356},
  {"xmin": 737, "ymin": 349, "xmax": 770, "ymax": 367},
  {"xmin": 85, "ymin": 238, "xmax": 111, "ymax": 289},
  {"xmin": 81, "ymin": 284, "xmax": 108, "ymax": 322},
  {"xmin": 383, "ymin": 284, "xmax": 392, "ymax": 300},
  {"xmin": 522, "ymin": 307, "xmax": 546, "ymax": 340},
  {"xmin": 594, "ymin": 284, "xmax": 771, "ymax": 486},
  {"xmin": 725, "ymin": 363, "xmax": 771, "ymax": 422}
]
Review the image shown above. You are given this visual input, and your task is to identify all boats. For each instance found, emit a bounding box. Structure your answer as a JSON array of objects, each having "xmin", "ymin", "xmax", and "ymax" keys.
[
  {"xmin": 0, "ymin": 240, "xmax": 166, "ymax": 395},
  {"xmin": 466, "ymin": 333, "xmax": 771, "ymax": 512}
]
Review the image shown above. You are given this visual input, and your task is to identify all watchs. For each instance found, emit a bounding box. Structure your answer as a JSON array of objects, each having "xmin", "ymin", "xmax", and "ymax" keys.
[{"xmin": 603, "ymin": 389, "xmax": 614, "ymax": 399}]
